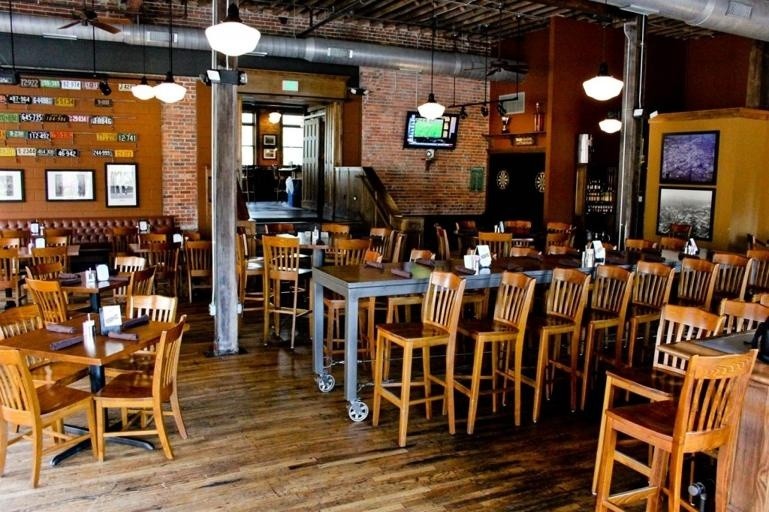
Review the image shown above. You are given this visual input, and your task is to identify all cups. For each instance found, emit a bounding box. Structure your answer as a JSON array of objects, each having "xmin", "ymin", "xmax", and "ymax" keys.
[
  {"xmin": 750, "ymin": 315, "xmax": 769, "ymax": 363},
  {"xmin": 297, "ymin": 232, "xmax": 306, "ymax": 245}
]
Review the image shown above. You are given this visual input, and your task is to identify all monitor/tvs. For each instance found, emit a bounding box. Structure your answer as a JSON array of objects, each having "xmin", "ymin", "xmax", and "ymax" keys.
[{"xmin": 404, "ymin": 111, "xmax": 460, "ymax": 149}]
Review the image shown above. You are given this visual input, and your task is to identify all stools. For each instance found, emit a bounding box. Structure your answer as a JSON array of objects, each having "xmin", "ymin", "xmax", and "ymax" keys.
[{"xmin": 276, "ymin": 167, "xmax": 296, "ymax": 201}]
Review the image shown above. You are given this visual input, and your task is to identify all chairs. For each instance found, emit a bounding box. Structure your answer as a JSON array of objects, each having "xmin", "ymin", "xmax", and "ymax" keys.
[
  {"xmin": 241, "ymin": 165, "xmax": 257, "ymax": 203},
  {"xmin": 271, "ymin": 164, "xmax": 285, "ymax": 193}
]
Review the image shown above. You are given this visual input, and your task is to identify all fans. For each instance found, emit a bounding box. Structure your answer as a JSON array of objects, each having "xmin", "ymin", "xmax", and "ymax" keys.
[
  {"xmin": 58, "ymin": 0, "xmax": 132, "ymax": 35},
  {"xmin": 463, "ymin": 0, "xmax": 530, "ymax": 76}
]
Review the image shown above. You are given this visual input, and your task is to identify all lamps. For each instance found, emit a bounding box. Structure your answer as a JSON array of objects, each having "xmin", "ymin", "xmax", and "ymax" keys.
[
  {"xmin": 599, "ymin": 117, "xmax": 622, "ymax": 133},
  {"xmin": 204, "ymin": 0, "xmax": 261, "ymax": 58},
  {"xmin": 582, "ymin": 0, "xmax": 624, "ymax": 102},
  {"xmin": 131, "ymin": 0, "xmax": 154, "ymax": 101},
  {"xmin": 448, "ymin": 96, "xmax": 518, "ymax": 120},
  {"xmin": 417, "ymin": 0, "xmax": 446, "ymax": 121},
  {"xmin": 153, "ymin": 0, "xmax": 186, "ymax": 104}
]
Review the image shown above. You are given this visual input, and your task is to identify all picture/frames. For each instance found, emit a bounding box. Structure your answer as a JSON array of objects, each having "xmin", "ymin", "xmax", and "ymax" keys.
[
  {"xmin": 660, "ymin": 130, "xmax": 719, "ymax": 184},
  {"xmin": 262, "ymin": 134, "xmax": 277, "ymax": 146},
  {"xmin": 45, "ymin": 168, "xmax": 96, "ymax": 203},
  {"xmin": 0, "ymin": 167, "xmax": 25, "ymax": 203},
  {"xmin": 105, "ymin": 161, "xmax": 140, "ymax": 207},
  {"xmin": 262, "ymin": 148, "xmax": 277, "ymax": 159},
  {"xmin": 652, "ymin": 186, "xmax": 717, "ymax": 242}
]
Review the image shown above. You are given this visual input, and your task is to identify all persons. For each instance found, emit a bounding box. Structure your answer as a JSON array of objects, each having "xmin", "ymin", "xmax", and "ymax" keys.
[{"xmin": 284, "ymin": 171, "xmax": 295, "ymax": 206}]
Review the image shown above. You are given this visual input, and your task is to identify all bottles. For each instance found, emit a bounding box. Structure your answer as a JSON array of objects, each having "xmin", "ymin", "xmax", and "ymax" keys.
[
  {"xmin": 82, "ymin": 313, "xmax": 96, "ymax": 337},
  {"xmin": 581, "ymin": 249, "xmax": 595, "ymax": 268},
  {"xmin": 39, "ymin": 223, "xmax": 45, "ymax": 236},
  {"xmin": 494, "ymin": 224, "xmax": 501, "ymax": 233},
  {"xmin": 683, "ymin": 241, "xmax": 694, "ymax": 255},
  {"xmin": 463, "ymin": 248, "xmax": 480, "ymax": 272},
  {"xmin": 532, "ymin": 102, "xmax": 545, "ymax": 131},
  {"xmin": 585, "ymin": 177, "xmax": 613, "ymax": 213},
  {"xmin": 27, "ymin": 238, "xmax": 35, "ymax": 255},
  {"xmin": 135, "ymin": 221, "xmax": 140, "ymax": 233},
  {"xmin": 85, "ymin": 267, "xmax": 96, "ymax": 282}
]
